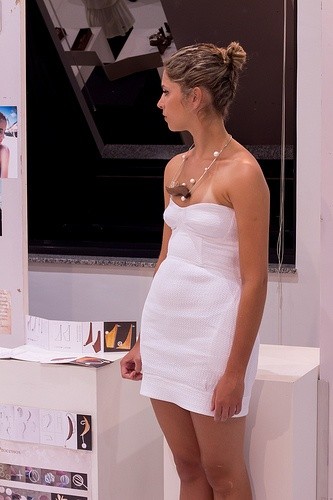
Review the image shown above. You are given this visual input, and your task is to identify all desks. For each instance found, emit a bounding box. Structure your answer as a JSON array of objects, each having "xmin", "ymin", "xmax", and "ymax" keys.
[
  {"xmin": 54, "ymin": 25, "xmax": 177, "ymax": 82},
  {"xmin": 0, "ymin": 345, "xmax": 321, "ymax": 500}
]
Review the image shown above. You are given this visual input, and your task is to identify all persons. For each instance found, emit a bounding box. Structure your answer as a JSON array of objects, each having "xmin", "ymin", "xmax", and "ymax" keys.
[
  {"xmin": 120, "ymin": 43, "xmax": 271, "ymax": 500},
  {"xmin": 0, "ymin": 112, "xmax": 11, "ymax": 178}
]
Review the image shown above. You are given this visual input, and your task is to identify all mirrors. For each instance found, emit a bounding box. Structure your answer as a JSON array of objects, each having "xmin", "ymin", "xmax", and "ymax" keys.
[{"xmin": 36, "ymin": 0, "xmax": 295, "ymax": 160}]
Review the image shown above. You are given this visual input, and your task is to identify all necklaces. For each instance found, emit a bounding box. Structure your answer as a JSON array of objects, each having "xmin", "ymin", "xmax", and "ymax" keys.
[{"xmin": 165, "ymin": 134, "xmax": 233, "ymax": 201}]
